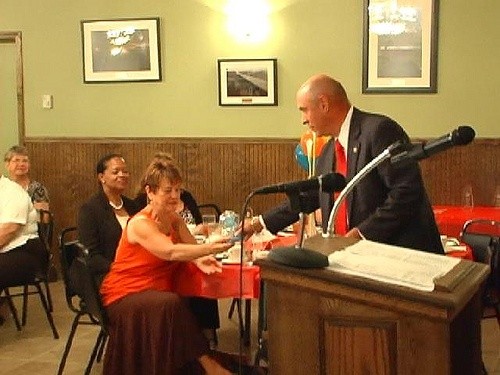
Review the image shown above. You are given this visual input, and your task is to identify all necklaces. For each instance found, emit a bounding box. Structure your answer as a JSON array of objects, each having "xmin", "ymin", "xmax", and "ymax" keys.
[{"xmin": 109, "ymin": 199, "xmax": 124, "ymax": 210}]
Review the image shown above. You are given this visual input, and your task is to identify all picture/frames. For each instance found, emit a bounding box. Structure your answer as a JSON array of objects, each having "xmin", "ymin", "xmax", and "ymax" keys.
[
  {"xmin": 362, "ymin": 0, "xmax": 440, "ymax": 93},
  {"xmin": 82, "ymin": 20, "xmax": 160, "ymax": 81},
  {"xmin": 218, "ymin": 60, "xmax": 277, "ymax": 106}
]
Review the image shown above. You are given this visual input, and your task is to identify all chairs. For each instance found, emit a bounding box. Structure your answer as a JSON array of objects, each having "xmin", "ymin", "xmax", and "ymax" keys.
[
  {"xmin": 460, "ymin": 219, "xmax": 500, "ymax": 320},
  {"xmin": 0, "ymin": 208, "xmax": 111, "ymax": 375}
]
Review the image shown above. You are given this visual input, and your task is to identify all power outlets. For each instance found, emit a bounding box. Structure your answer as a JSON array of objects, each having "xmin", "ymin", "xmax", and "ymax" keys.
[{"xmin": 43, "ymin": 95, "xmax": 51, "ymax": 108}]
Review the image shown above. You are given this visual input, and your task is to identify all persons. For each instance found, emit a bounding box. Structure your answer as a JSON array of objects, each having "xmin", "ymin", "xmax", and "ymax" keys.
[
  {"xmin": 98, "ymin": 153, "xmax": 235, "ymax": 375},
  {"xmin": 234, "ymin": 75, "xmax": 447, "ymax": 256},
  {"xmin": 134, "ymin": 152, "xmax": 203, "ymax": 225},
  {"xmin": 0, "ymin": 171, "xmax": 49, "ymax": 327},
  {"xmin": 78, "ymin": 154, "xmax": 138, "ymax": 330},
  {"xmin": 3, "ymin": 146, "xmax": 50, "ymax": 242}
]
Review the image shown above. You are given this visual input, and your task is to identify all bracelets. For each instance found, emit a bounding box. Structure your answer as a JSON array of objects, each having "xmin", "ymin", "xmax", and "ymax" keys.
[{"xmin": 251, "ymin": 217, "xmax": 261, "ymax": 235}]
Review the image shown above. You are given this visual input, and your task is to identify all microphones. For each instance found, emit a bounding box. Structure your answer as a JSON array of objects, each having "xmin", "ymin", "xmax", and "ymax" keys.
[
  {"xmin": 253, "ymin": 173, "xmax": 346, "ymax": 194},
  {"xmin": 390, "ymin": 126, "xmax": 475, "ymax": 168}
]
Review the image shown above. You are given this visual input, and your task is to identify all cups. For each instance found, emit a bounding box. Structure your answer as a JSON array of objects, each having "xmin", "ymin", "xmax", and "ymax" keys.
[
  {"xmin": 202, "ymin": 215, "xmax": 216, "ymax": 243},
  {"xmin": 207, "ymin": 223, "xmax": 222, "ymax": 243},
  {"xmin": 231, "ymin": 226, "xmax": 242, "ymax": 241},
  {"xmin": 222, "ymin": 228, "xmax": 234, "ymax": 245}
]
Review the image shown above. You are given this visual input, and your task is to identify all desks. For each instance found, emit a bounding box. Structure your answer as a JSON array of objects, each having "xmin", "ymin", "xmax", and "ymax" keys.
[
  {"xmin": 176, "ymin": 220, "xmax": 266, "ymax": 375},
  {"xmin": 426, "ymin": 203, "xmax": 500, "ymax": 239}
]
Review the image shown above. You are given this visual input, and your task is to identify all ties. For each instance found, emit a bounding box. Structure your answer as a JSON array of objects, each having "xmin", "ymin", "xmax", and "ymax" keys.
[{"xmin": 334, "ymin": 140, "xmax": 348, "ymax": 236}]
